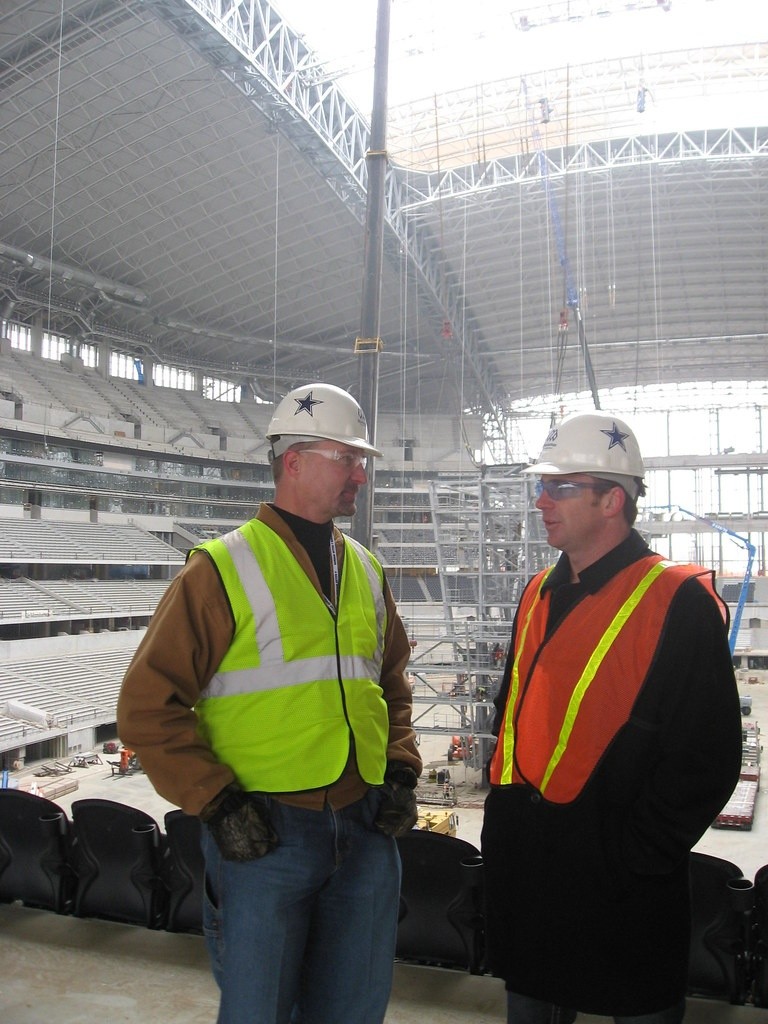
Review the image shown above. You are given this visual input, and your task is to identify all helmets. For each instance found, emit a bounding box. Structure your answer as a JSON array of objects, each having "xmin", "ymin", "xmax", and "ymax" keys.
[
  {"xmin": 520, "ymin": 414, "xmax": 647, "ymax": 480},
  {"xmin": 265, "ymin": 382, "xmax": 385, "ymax": 458}
]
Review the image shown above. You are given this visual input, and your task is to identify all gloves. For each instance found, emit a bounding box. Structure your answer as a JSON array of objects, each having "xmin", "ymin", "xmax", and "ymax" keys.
[
  {"xmin": 374, "ymin": 781, "xmax": 418, "ymax": 840},
  {"xmin": 207, "ymin": 791, "xmax": 270, "ymax": 863}
]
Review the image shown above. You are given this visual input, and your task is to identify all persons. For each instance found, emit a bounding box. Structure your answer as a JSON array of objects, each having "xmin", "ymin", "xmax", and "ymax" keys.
[
  {"xmin": 480, "ymin": 412, "xmax": 744, "ymax": 1024},
  {"xmin": 115, "ymin": 383, "xmax": 422, "ymax": 1024}
]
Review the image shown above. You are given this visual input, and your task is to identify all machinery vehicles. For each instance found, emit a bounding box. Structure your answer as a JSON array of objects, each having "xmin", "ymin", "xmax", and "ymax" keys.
[{"xmin": 638, "ymin": 502, "xmax": 758, "ymax": 717}]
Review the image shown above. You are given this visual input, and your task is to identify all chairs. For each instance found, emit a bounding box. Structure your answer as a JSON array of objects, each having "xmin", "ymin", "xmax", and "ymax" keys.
[
  {"xmin": 0, "ymin": 787, "xmax": 72, "ymax": 916},
  {"xmin": 72, "ymin": 799, "xmax": 165, "ymax": 932},
  {"xmin": 161, "ymin": 809, "xmax": 204, "ymax": 935},
  {"xmin": 395, "ymin": 830, "xmax": 488, "ymax": 977},
  {"xmin": 747, "ymin": 865, "xmax": 768, "ymax": 1009},
  {"xmin": 685, "ymin": 851, "xmax": 754, "ymax": 1007}
]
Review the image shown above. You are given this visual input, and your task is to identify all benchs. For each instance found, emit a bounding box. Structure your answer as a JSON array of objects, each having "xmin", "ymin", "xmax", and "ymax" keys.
[
  {"xmin": 0, "ymin": 517, "xmax": 186, "ymax": 742},
  {"xmin": 0, "ymin": 350, "xmax": 278, "ymax": 440}
]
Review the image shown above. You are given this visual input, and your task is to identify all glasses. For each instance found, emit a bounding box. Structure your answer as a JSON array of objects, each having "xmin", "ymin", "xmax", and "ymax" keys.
[
  {"xmin": 297, "ymin": 449, "xmax": 368, "ymax": 469},
  {"xmin": 532, "ymin": 480, "xmax": 610, "ymax": 502}
]
